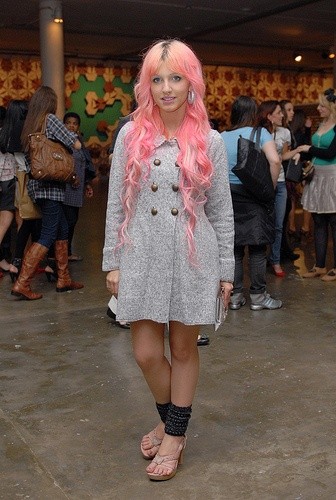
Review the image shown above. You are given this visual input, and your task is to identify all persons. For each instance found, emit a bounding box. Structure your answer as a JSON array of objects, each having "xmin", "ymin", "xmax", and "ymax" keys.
[
  {"xmin": 217, "ymin": 96, "xmax": 281, "ymax": 313},
  {"xmin": 102, "ymin": 41, "xmax": 235, "ymax": 482},
  {"xmin": 259, "ymin": 89, "xmax": 336, "ymax": 281},
  {"xmin": 106, "ymin": 100, "xmax": 210, "ymax": 344},
  {"xmin": 0, "ymin": 85, "xmax": 95, "ymax": 300}
]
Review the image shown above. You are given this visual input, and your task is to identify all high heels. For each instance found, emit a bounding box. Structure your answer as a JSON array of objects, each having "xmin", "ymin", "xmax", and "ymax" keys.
[
  {"xmin": 288, "ymin": 229, "xmax": 301, "ymax": 240},
  {"xmin": 272, "ymin": 265, "xmax": 287, "ymax": 277},
  {"xmin": 0, "ymin": 264, "xmax": 10, "ymax": 272},
  {"xmin": 45, "ymin": 263, "xmax": 58, "ymax": 282},
  {"xmin": 299, "ymin": 229, "xmax": 313, "ymax": 240},
  {"xmin": 141, "ymin": 423, "xmax": 165, "ymax": 460},
  {"xmin": 9, "ymin": 258, "xmax": 22, "ymax": 283},
  {"xmin": 107, "ymin": 307, "xmax": 131, "ymax": 329},
  {"xmin": 147, "ymin": 433, "xmax": 187, "ymax": 480}
]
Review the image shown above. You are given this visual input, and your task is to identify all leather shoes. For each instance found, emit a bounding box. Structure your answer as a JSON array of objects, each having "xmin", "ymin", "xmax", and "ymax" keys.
[
  {"xmin": 302, "ymin": 267, "xmax": 326, "ymax": 278},
  {"xmin": 321, "ymin": 269, "xmax": 336, "ymax": 281},
  {"xmin": 197, "ymin": 333, "xmax": 209, "ymax": 345}
]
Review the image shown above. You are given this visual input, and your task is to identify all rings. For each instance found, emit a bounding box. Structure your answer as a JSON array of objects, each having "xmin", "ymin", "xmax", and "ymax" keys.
[{"xmin": 108, "ymin": 287, "xmax": 111, "ymax": 289}]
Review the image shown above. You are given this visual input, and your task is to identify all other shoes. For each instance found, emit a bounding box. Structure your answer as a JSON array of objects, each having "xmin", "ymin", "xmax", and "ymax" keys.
[
  {"xmin": 266, "ymin": 257, "xmax": 272, "ymax": 267},
  {"xmin": 68, "ymin": 255, "xmax": 83, "ymax": 261},
  {"xmin": 286, "ymin": 253, "xmax": 300, "ymax": 261}
]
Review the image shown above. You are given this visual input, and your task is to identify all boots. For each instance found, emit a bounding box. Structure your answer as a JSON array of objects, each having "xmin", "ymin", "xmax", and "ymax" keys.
[
  {"xmin": 11, "ymin": 242, "xmax": 50, "ymax": 300},
  {"xmin": 55, "ymin": 240, "xmax": 84, "ymax": 292}
]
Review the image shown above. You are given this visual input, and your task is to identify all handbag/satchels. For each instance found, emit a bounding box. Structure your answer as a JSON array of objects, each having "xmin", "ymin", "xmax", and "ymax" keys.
[
  {"xmin": 27, "ymin": 113, "xmax": 75, "ymax": 183},
  {"xmin": 231, "ymin": 126, "xmax": 275, "ymax": 204},
  {"xmin": 16, "ymin": 170, "xmax": 43, "ymax": 219},
  {"xmin": 285, "ymin": 159, "xmax": 302, "ymax": 183},
  {"xmin": 300, "ymin": 161, "xmax": 316, "ymax": 184}
]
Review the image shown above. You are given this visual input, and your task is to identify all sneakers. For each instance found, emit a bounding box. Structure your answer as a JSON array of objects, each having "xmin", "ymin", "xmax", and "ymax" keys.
[
  {"xmin": 250, "ymin": 290, "xmax": 283, "ymax": 311},
  {"xmin": 229, "ymin": 293, "xmax": 246, "ymax": 309}
]
